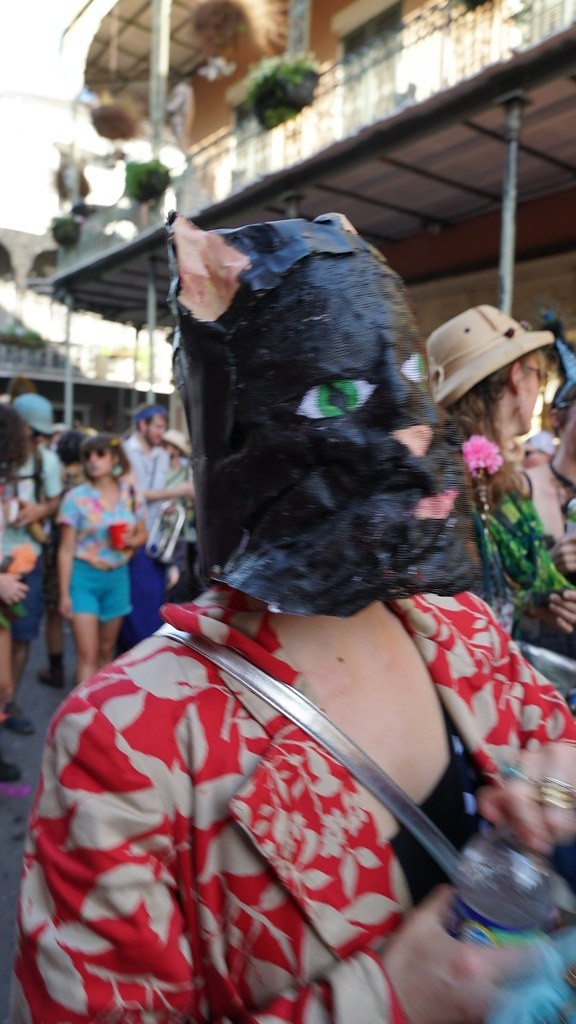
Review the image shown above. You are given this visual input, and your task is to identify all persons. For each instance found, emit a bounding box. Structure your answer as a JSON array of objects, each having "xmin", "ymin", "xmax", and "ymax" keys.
[{"xmin": 0, "ymin": 211, "xmax": 576, "ymax": 1024}]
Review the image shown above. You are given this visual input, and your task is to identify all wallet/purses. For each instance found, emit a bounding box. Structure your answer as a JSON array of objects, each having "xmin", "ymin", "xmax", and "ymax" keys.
[{"xmin": 109, "ymin": 524, "xmax": 130, "ymax": 548}]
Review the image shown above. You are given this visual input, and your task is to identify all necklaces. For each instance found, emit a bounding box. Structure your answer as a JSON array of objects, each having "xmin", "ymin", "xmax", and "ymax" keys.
[{"xmin": 549, "ymin": 459, "xmax": 573, "ymax": 488}]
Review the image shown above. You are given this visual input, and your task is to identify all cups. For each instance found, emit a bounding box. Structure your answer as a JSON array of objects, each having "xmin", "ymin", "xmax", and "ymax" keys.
[
  {"xmin": 447, "ymin": 823, "xmax": 551, "ymax": 949},
  {"xmin": 108, "ymin": 522, "xmax": 128, "ymax": 549}
]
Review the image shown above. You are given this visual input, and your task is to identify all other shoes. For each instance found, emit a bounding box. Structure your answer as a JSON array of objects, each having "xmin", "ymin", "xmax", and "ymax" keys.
[
  {"xmin": 2, "ymin": 705, "xmax": 34, "ymax": 734},
  {"xmin": 36, "ymin": 669, "xmax": 65, "ymax": 688},
  {"xmin": 0, "ymin": 756, "xmax": 23, "ymax": 782}
]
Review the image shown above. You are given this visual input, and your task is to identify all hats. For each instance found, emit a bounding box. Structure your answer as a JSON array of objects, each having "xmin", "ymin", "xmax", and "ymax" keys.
[
  {"xmin": 525, "ymin": 433, "xmax": 555, "ymax": 454},
  {"xmin": 161, "ymin": 428, "xmax": 192, "ymax": 457},
  {"xmin": 424, "ymin": 304, "xmax": 555, "ymax": 408},
  {"xmin": 14, "ymin": 391, "xmax": 56, "ymax": 434}
]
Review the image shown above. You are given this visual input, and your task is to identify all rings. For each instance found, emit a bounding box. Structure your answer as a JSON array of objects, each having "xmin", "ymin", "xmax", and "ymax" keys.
[{"xmin": 542, "ymin": 779, "xmax": 576, "ymax": 811}]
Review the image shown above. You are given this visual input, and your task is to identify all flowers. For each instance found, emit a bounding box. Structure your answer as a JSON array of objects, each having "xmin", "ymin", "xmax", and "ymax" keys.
[{"xmin": 462, "ymin": 432, "xmax": 508, "ymax": 482}]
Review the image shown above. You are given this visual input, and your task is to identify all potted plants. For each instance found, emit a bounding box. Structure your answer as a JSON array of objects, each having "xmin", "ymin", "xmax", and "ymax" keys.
[
  {"xmin": 49, "ymin": 214, "xmax": 84, "ymax": 251},
  {"xmin": 120, "ymin": 157, "xmax": 173, "ymax": 202},
  {"xmin": 227, "ymin": 50, "xmax": 320, "ymax": 129}
]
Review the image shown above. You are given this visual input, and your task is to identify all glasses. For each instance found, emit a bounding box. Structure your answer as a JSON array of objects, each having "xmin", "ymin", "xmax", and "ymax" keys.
[
  {"xmin": 80, "ymin": 447, "xmax": 109, "ymax": 461},
  {"xmin": 525, "ymin": 450, "xmax": 539, "ymax": 456},
  {"xmin": 524, "ymin": 365, "xmax": 550, "ymax": 385}
]
